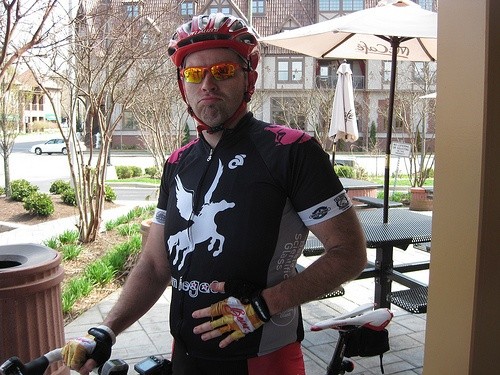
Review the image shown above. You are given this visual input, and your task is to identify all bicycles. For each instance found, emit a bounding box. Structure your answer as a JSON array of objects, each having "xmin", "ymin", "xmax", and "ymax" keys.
[{"xmin": 0, "ymin": 301, "xmax": 396, "ymax": 375}]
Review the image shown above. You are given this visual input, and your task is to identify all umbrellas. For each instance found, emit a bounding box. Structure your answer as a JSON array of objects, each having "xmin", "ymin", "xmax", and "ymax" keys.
[
  {"xmin": 328, "ymin": 59, "xmax": 358, "ymax": 168},
  {"xmin": 259, "ymin": 0, "xmax": 438, "ymax": 225}
]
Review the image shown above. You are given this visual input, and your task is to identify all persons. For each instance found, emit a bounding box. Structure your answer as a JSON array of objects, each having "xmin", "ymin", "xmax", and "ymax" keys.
[{"xmin": 63, "ymin": 13, "xmax": 367, "ymax": 375}]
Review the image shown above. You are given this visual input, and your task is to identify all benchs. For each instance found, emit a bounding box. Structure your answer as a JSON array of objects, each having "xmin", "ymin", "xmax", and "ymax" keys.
[
  {"xmin": 385, "ymin": 286, "xmax": 428, "ymax": 314},
  {"xmin": 352, "ymin": 196, "xmax": 403, "ymax": 208},
  {"xmin": 295, "ymin": 264, "xmax": 345, "ymax": 300},
  {"xmin": 413, "ymin": 242, "xmax": 431, "ymax": 253}
]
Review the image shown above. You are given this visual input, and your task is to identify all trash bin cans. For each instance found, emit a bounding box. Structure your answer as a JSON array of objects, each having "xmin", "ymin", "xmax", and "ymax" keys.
[
  {"xmin": 0, "ymin": 243, "xmax": 66, "ymax": 375},
  {"xmin": 344, "ymin": 185, "xmax": 377, "ymax": 205},
  {"xmin": 409, "ymin": 187, "xmax": 433, "ymax": 212}
]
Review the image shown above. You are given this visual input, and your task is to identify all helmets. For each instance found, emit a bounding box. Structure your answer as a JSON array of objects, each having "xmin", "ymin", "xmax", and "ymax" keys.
[{"xmin": 167, "ymin": 12, "xmax": 259, "ymax": 72}]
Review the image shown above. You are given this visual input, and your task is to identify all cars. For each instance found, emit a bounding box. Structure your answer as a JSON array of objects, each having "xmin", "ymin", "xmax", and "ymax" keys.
[{"xmin": 30, "ymin": 136, "xmax": 87, "ymax": 155}]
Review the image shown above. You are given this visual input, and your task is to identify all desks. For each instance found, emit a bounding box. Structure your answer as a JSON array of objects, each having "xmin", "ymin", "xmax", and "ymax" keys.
[
  {"xmin": 339, "ymin": 178, "xmax": 383, "ymax": 193},
  {"xmin": 303, "ymin": 209, "xmax": 432, "ymax": 309}
]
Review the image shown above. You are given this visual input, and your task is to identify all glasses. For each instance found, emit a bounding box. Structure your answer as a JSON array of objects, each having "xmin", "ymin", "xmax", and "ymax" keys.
[{"xmin": 179, "ymin": 63, "xmax": 251, "ymax": 84}]
